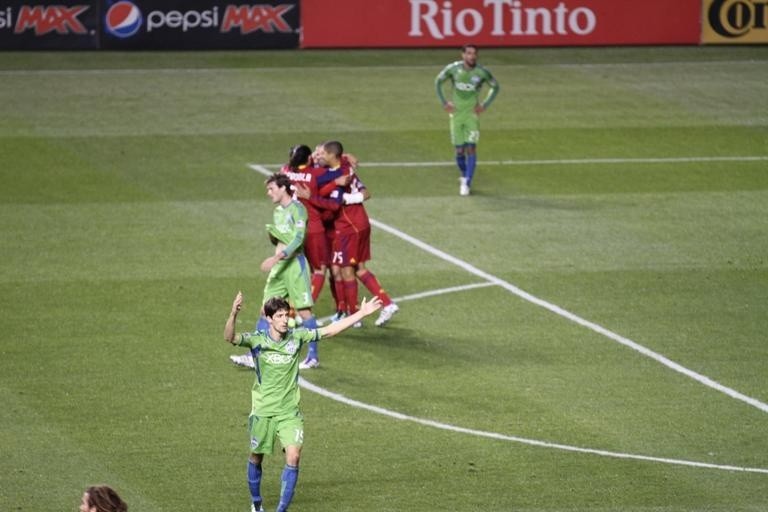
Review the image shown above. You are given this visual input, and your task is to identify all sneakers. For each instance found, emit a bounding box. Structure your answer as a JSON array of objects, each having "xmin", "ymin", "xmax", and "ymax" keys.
[
  {"xmin": 375, "ymin": 303, "xmax": 400, "ymax": 328},
  {"xmin": 458, "ymin": 176, "xmax": 470, "ymax": 196},
  {"xmin": 329, "ymin": 312, "xmax": 345, "ymax": 321},
  {"xmin": 296, "ymin": 313, "xmax": 324, "ymax": 326},
  {"xmin": 250, "ymin": 503, "xmax": 265, "ymax": 512},
  {"xmin": 298, "ymin": 356, "xmax": 320, "ymax": 370},
  {"xmin": 225, "ymin": 353, "xmax": 256, "ymax": 369},
  {"xmin": 352, "ymin": 320, "xmax": 362, "ymax": 328}
]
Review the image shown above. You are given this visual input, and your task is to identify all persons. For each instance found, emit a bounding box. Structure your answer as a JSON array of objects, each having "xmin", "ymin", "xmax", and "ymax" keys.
[
  {"xmin": 228, "ymin": 171, "xmax": 322, "ymax": 371},
  {"xmin": 434, "ymin": 42, "xmax": 501, "ymax": 200},
  {"xmin": 277, "ymin": 140, "xmax": 401, "ymax": 332},
  {"xmin": 221, "ymin": 291, "xmax": 384, "ymax": 511},
  {"xmin": 82, "ymin": 483, "xmax": 130, "ymax": 511}
]
